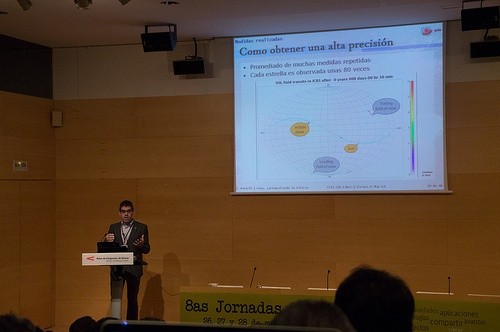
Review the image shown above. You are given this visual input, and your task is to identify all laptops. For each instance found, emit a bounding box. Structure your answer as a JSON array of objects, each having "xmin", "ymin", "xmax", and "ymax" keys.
[{"xmin": 97, "ymin": 242, "xmax": 121, "ymax": 254}]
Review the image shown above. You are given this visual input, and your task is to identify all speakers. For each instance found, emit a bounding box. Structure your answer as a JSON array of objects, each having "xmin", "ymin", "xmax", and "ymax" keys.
[
  {"xmin": 470, "ymin": 40, "xmax": 500, "ymax": 58},
  {"xmin": 173, "ymin": 59, "xmax": 205, "ymax": 76},
  {"xmin": 141, "ymin": 32, "xmax": 176, "ymax": 53},
  {"xmin": 461, "ymin": 6, "xmax": 500, "ymax": 31},
  {"xmin": 52, "ymin": 110, "xmax": 62, "ymax": 126}
]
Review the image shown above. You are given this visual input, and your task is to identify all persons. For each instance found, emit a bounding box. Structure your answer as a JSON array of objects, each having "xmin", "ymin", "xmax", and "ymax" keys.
[
  {"xmin": 0, "ymin": 315, "xmax": 127, "ymax": 332},
  {"xmin": 333, "ymin": 267, "xmax": 415, "ymax": 332},
  {"xmin": 104, "ymin": 200, "xmax": 150, "ymax": 320}
]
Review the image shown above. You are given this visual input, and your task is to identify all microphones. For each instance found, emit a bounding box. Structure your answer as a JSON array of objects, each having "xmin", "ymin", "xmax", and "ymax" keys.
[
  {"xmin": 250, "ymin": 267, "xmax": 256, "ymax": 287},
  {"xmin": 448, "ymin": 276, "xmax": 451, "ymax": 294},
  {"xmin": 327, "ymin": 270, "xmax": 331, "ymax": 289}
]
